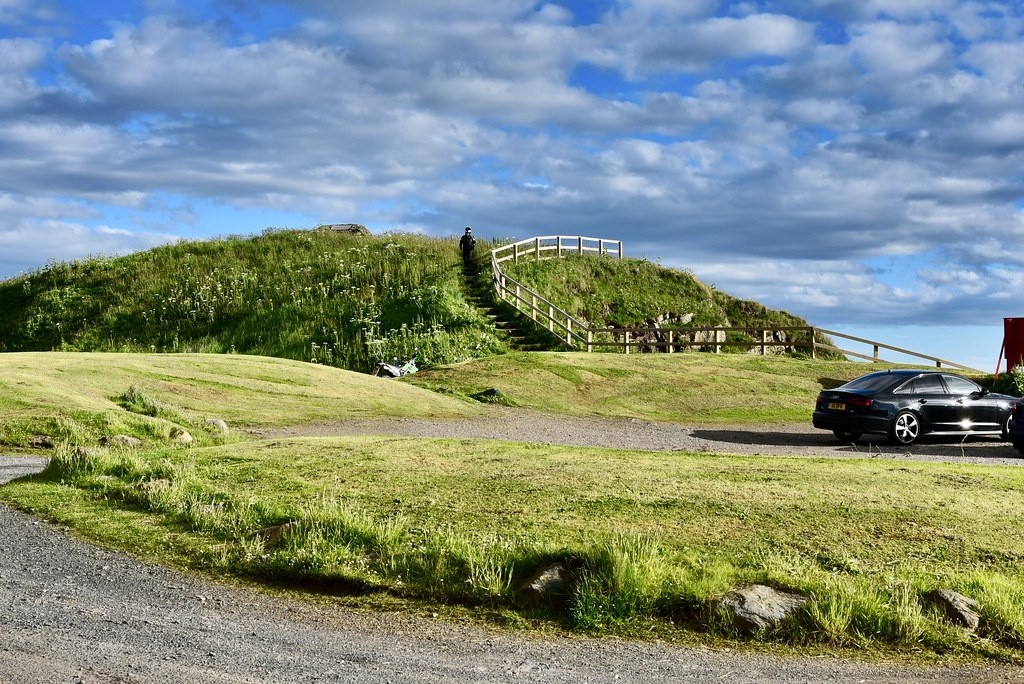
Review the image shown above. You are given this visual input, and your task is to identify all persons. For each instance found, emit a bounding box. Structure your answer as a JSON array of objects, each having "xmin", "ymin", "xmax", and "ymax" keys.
[{"xmin": 460, "ymin": 226, "xmax": 476, "ymax": 268}]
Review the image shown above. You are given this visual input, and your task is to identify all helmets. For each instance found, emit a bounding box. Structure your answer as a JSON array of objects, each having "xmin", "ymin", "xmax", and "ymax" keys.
[{"xmin": 465, "ymin": 227, "xmax": 472, "ymax": 233}]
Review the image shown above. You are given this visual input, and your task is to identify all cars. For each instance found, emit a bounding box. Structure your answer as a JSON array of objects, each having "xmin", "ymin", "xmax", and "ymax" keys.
[
  {"xmin": 1006, "ymin": 393, "xmax": 1024, "ymax": 457},
  {"xmin": 811, "ymin": 369, "xmax": 1021, "ymax": 447}
]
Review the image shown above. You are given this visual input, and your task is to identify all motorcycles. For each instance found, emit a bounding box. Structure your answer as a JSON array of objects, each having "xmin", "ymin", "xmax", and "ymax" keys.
[{"xmin": 371, "ymin": 346, "xmax": 421, "ymax": 380}]
